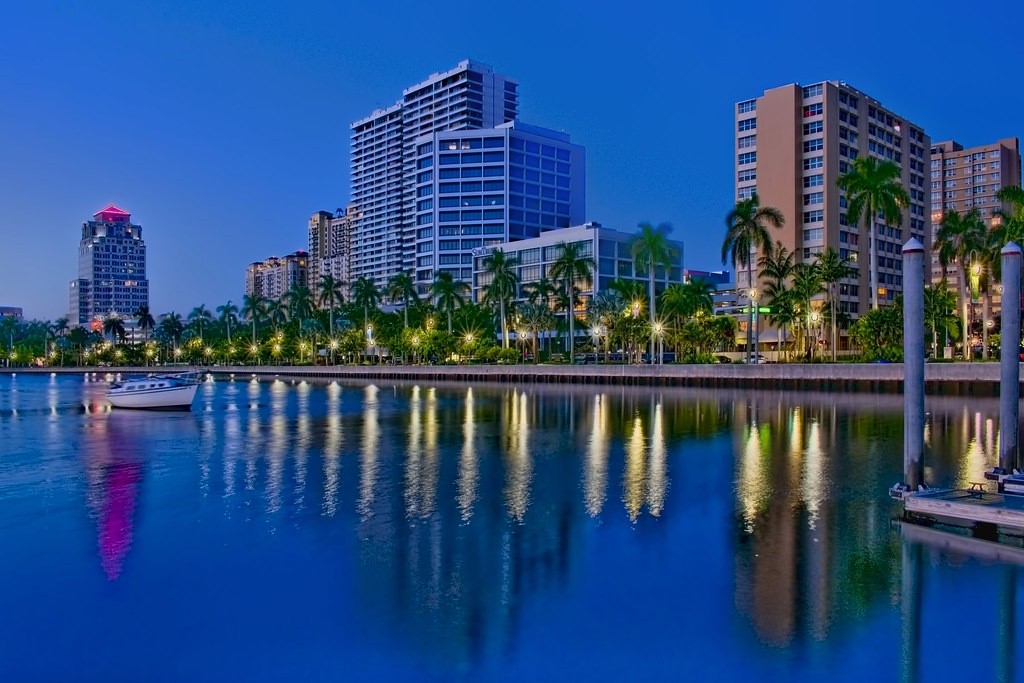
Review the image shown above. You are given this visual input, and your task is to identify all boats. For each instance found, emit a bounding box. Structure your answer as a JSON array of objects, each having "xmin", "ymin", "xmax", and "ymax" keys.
[{"xmin": 95, "ymin": 368, "xmax": 206, "ymax": 413}]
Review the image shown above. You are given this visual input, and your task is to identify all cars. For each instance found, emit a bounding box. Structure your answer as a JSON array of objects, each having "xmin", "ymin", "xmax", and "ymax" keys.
[
  {"xmin": 709, "ymin": 355, "xmax": 731, "ymax": 364},
  {"xmin": 742, "ymin": 354, "xmax": 767, "ymax": 364},
  {"xmin": 625, "ymin": 352, "xmax": 656, "ymax": 362}
]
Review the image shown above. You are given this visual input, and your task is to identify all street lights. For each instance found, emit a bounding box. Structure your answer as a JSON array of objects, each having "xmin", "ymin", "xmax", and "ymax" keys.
[
  {"xmin": 146, "ymin": 348, "xmax": 153, "ymax": 369},
  {"xmin": 631, "ymin": 293, "xmax": 640, "ymax": 367},
  {"xmin": 519, "ymin": 329, "xmax": 528, "ymax": 364},
  {"xmin": 425, "ymin": 312, "xmax": 434, "ymax": 365},
  {"xmin": 465, "ymin": 332, "xmax": 472, "ymax": 363},
  {"xmin": 206, "ymin": 346, "xmax": 211, "ymax": 366},
  {"xmin": 274, "ymin": 333, "xmax": 282, "ymax": 367},
  {"xmin": 808, "ymin": 308, "xmax": 819, "ymax": 360},
  {"xmin": 251, "ymin": 346, "xmax": 257, "ymax": 366},
  {"xmin": 749, "ymin": 289, "xmax": 762, "ymax": 364},
  {"xmin": 365, "ymin": 319, "xmax": 376, "ymax": 367},
  {"xmin": 413, "ymin": 338, "xmax": 419, "ymax": 366},
  {"xmin": 332, "ymin": 339, "xmax": 338, "ymax": 366},
  {"xmin": 593, "ymin": 321, "xmax": 601, "ymax": 364},
  {"xmin": 653, "ymin": 322, "xmax": 663, "ymax": 364},
  {"xmin": 967, "ymin": 249, "xmax": 980, "ymax": 362},
  {"xmin": 300, "ymin": 343, "xmax": 305, "ymax": 364}
]
